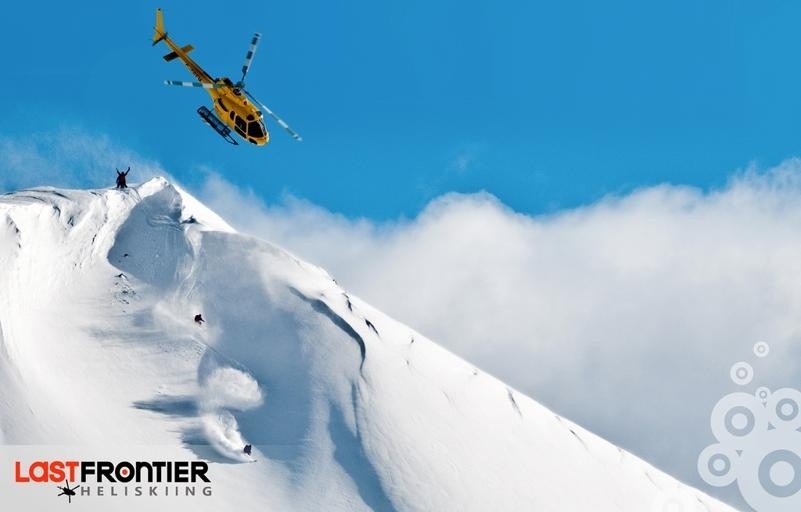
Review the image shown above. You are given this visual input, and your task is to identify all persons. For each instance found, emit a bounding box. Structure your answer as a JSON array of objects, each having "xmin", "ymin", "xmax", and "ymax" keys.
[
  {"xmin": 243, "ymin": 443, "xmax": 252, "ymax": 456},
  {"xmin": 194, "ymin": 314, "xmax": 207, "ymax": 326},
  {"xmin": 116, "ymin": 165, "xmax": 131, "ymax": 190}
]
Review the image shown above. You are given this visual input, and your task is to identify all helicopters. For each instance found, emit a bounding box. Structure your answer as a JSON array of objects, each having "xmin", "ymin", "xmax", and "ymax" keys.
[{"xmin": 153, "ymin": 7, "xmax": 303, "ymax": 147}]
